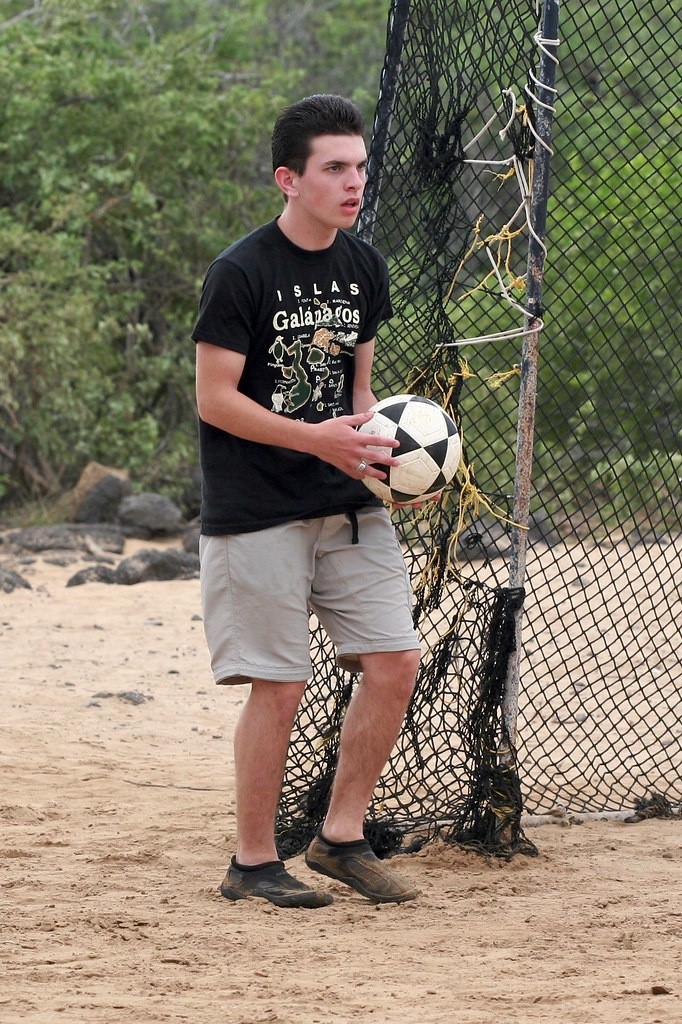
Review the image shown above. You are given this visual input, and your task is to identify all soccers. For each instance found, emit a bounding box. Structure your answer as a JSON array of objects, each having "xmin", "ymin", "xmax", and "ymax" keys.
[{"xmin": 355, "ymin": 393, "xmax": 460, "ymax": 504}]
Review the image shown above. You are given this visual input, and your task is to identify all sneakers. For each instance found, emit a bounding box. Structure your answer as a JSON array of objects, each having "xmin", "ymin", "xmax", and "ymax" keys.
[
  {"xmin": 306, "ymin": 830, "xmax": 417, "ymax": 903},
  {"xmin": 221, "ymin": 854, "xmax": 334, "ymax": 908}
]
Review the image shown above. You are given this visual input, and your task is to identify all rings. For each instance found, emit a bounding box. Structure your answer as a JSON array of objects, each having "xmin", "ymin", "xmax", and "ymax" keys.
[{"xmin": 355, "ymin": 461, "xmax": 366, "ymax": 472}]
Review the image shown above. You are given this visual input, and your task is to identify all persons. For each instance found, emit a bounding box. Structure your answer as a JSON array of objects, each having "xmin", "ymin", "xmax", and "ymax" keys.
[{"xmin": 191, "ymin": 95, "xmax": 441, "ymax": 910}]
